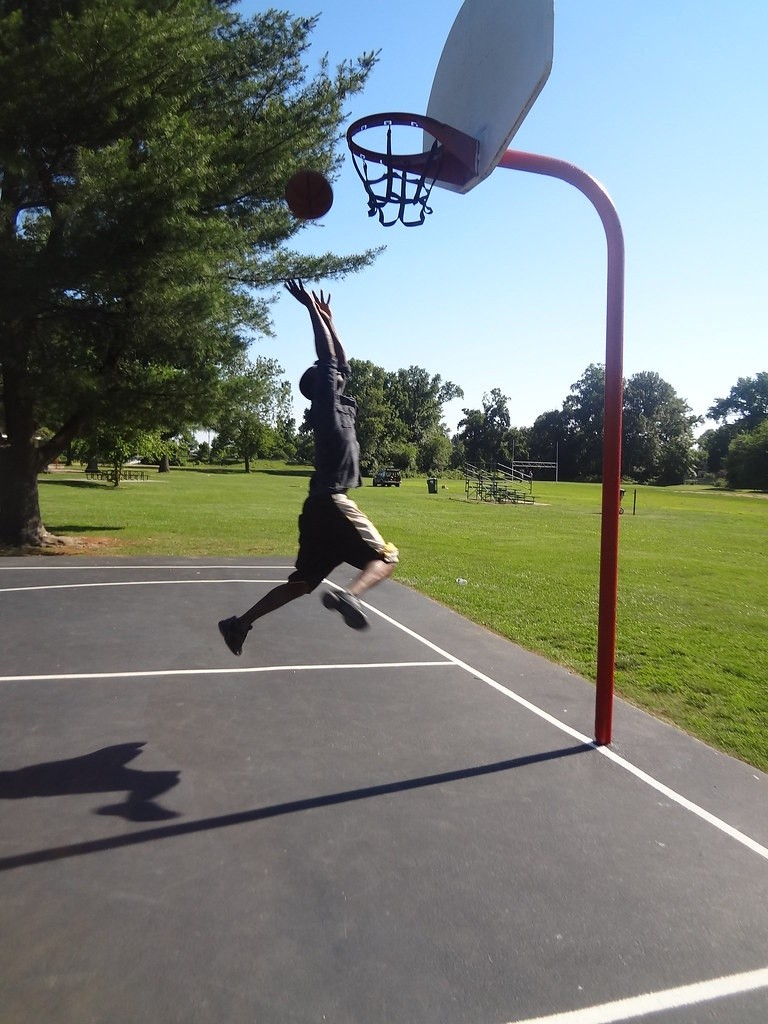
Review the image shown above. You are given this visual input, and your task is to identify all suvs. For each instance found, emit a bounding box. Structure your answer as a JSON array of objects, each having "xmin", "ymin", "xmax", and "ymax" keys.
[{"xmin": 373, "ymin": 467, "xmax": 402, "ymax": 488}]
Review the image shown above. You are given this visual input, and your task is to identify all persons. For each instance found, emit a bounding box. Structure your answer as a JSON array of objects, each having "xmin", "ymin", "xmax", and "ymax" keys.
[{"xmin": 215, "ymin": 277, "xmax": 403, "ymax": 656}]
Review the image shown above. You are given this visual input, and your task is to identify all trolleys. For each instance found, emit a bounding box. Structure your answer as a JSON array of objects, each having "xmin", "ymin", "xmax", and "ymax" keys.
[{"xmin": 618, "ymin": 489, "xmax": 626, "ymax": 514}]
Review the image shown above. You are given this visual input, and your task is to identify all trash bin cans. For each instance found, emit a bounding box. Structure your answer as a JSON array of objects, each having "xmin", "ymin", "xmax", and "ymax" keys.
[{"xmin": 427, "ymin": 478, "xmax": 438, "ymax": 494}]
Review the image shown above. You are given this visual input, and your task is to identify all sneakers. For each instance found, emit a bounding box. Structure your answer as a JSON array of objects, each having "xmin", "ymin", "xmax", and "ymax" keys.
[
  {"xmin": 218, "ymin": 615, "xmax": 252, "ymax": 657},
  {"xmin": 322, "ymin": 590, "xmax": 371, "ymax": 632}
]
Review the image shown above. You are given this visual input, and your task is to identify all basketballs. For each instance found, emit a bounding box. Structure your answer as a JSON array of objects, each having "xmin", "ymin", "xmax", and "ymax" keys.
[{"xmin": 285, "ymin": 171, "xmax": 333, "ymax": 219}]
